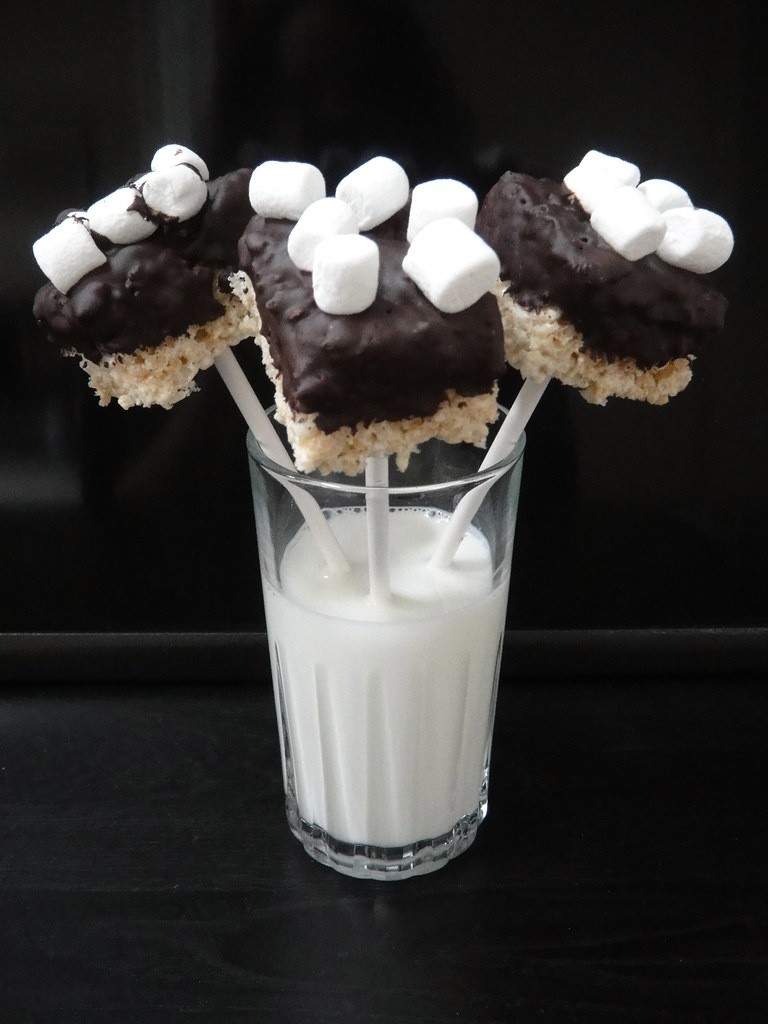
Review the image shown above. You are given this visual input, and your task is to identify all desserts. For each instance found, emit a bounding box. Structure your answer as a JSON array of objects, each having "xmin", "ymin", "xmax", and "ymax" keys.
[{"xmin": 33, "ymin": 142, "xmax": 734, "ymax": 480}]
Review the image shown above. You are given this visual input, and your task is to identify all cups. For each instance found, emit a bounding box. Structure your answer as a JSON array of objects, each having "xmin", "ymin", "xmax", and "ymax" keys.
[{"xmin": 246, "ymin": 397, "xmax": 527, "ymax": 881}]
